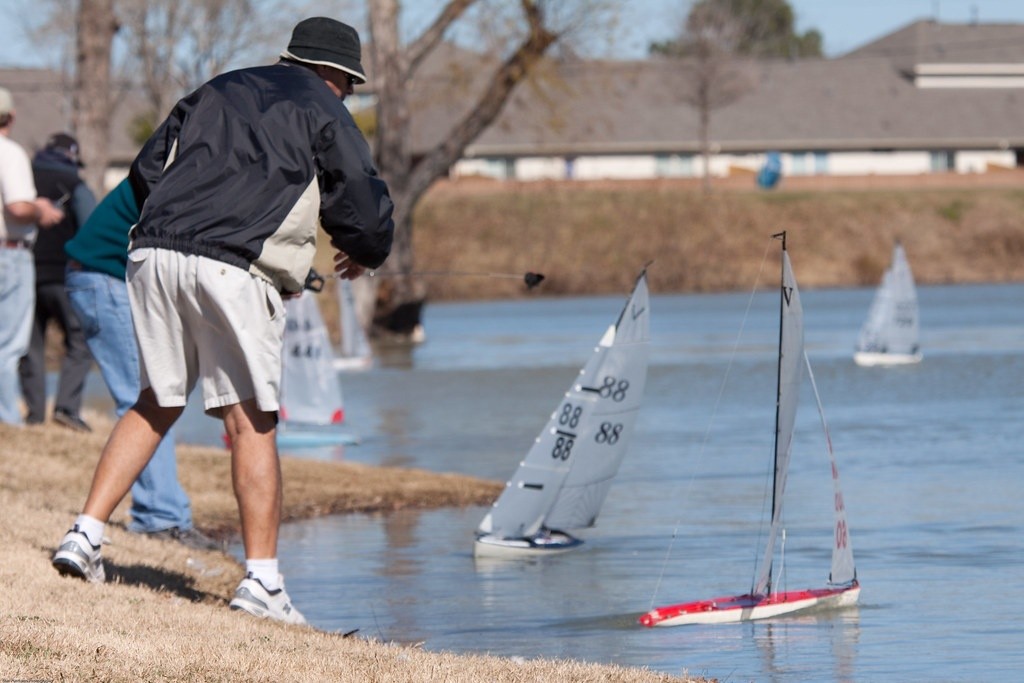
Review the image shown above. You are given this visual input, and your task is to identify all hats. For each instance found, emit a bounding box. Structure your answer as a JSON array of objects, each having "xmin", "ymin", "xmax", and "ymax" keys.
[
  {"xmin": 49, "ymin": 133, "xmax": 86, "ymax": 168},
  {"xmin": 279, "ymin": 15, "xmax": 367, "ymax": 84},
  {"xmin": 0, "ymin": 87, "xmax": 14, "ymax": 123}
]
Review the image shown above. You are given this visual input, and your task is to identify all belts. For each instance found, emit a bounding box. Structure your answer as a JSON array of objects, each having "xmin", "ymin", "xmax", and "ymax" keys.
[{"xmin": 0, "ymin": 239, "xmax": 30, "ymax": 248}]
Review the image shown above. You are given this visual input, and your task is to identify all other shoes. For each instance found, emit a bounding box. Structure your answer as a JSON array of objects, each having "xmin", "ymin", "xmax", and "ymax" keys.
[
  {"xmin": 54, "ymin": 408, "xmax": 93, "ymax": 433},
  {"xmin": 22, "ymin": 413, "xmax": 45, "ymax": 424},
  {"xmin": 149, "ymin": 526, "xmax": 221, "ymax": 551}
]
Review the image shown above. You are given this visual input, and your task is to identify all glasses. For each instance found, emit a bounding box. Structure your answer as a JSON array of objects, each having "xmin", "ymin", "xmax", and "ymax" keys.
[{"xmin": 340, "ymin": 69, "xmax": 358, "ymax": 86}]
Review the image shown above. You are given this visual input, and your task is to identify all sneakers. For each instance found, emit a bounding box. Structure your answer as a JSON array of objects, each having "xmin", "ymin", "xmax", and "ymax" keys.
[
  {"xmin": 52, "ymin": 523, "xmax": 105, "ymax": 585},
  {"xmin": 229, "ymin": 570, "xmax": 306, "ymax": 625}
]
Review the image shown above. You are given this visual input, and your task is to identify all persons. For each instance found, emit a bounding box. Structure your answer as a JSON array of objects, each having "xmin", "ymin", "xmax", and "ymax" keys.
[{"xmin": 1, "ymin": 17, "xmax": 394, "ymax": 626}]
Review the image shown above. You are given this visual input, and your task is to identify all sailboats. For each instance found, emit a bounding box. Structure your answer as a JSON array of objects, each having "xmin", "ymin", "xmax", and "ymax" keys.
[
  {"xmin": 470, "ymin": 257, "xmax": 657, "ymax": 568},
  {"xmin": 283, "ymin": 290, "xmax": 375, "ymax": 428},
  {"xmin": 854, "ymin": 240, "xmax": 925, "ymax": 366},
  {"xmin": 636, "ymin": 231, "xmax": 861, "ymax": 628}
]
